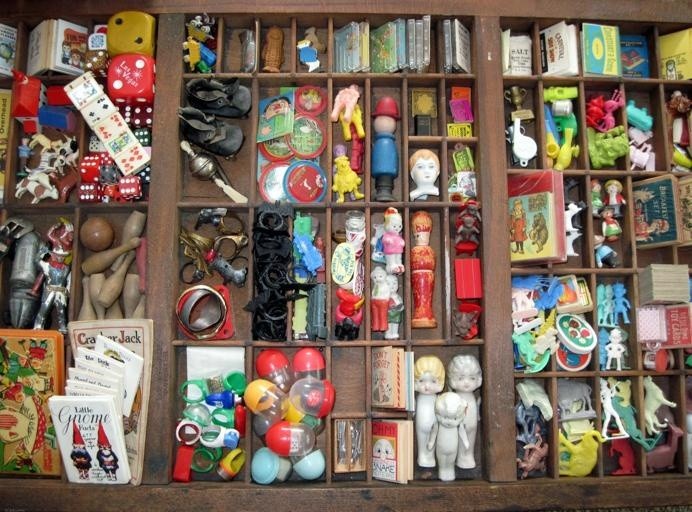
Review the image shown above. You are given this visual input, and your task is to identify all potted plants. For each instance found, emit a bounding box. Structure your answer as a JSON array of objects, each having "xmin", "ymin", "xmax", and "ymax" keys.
[{"xmin": 334, "ymin": 419, "xmax": 367, "ymax": 474}]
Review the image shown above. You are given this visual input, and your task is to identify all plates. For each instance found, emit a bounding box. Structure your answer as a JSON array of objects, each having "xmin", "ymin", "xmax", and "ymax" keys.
[{"xmin": 0, "ymin": 1, "xmax": 692, "ymax": 512}]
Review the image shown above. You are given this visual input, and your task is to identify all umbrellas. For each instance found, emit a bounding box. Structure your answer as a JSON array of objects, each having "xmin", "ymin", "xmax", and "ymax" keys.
[
  {"xmin": 185, "ymin": 77, "xmax": 251, "ymax": 118},
  {"xmin": 176, "ymin": 106, "xmax": 243, "ymax": 156}
]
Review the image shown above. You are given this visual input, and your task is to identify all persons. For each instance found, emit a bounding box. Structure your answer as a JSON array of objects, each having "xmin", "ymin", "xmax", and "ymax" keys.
[
  {"xmin": 414, "ymin": 355, "xmax": 445, "ymax": 468},
  {"xmin": 370, "ymin": 266, "xmax": 391, "ymax": 332},
  {"xmin": 409, "ymin": 149, "xmax": 439, "ymax": 203},
  {"xmin": 604, "ymin": 179, "xmax": 628, "ymax": 219},
  {"xmin": 28, "ymin": 216, "xmax": 76, "ymax": 336},
  {"xmin": 426, "ymin": 392, "xmax": 469, "ymax": 481},
  {"xmin": 592, "ymin": 235, "xmax": 618, "ymax": 268},
  {"xmin": 590, "ymin": 178, "xmax": 604, "ymax": 217},
  {"xmin": 383, "ymin": 275, "xmax": 405, "ymax": 339},
  {"xmin": 383, "ymin": 206, "xmax": 406, "ymax": 276},
  {"xmin": 447, "ymin": 355, "xmax": 483, "ymax": 469},
  {"xmin": 598, "ymin": 205, "xmax": 623, "ymax": 242}
]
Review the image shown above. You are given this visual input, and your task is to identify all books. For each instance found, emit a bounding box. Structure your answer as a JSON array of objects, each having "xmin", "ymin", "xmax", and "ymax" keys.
[
  {"xmin": 632, "ymin": 175, "xmax": 684, "ymax": 250},
  {"xmin": 49, "ymin": 334, "xmax": 144, "ymax": 485},
  {"xmin": 373, "ymin": 418, "xmax": 414, "ymax": 485},
  {"xmin": 508, "ymin": 169, "xmax": 568, "ymax": 265},
  {"xmin": 371, "ymin": 346, "xmax": 415, "ymax": 413}
]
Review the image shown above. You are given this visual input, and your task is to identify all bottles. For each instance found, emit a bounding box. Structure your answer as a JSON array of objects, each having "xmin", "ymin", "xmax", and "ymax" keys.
[
  {"xmin": 73, "ymin": 420, "xmax": 84, "ymax": 444},
  {"xmin": 98, "ymin": 420, "xmax": 109, "ymax": 445}
]
